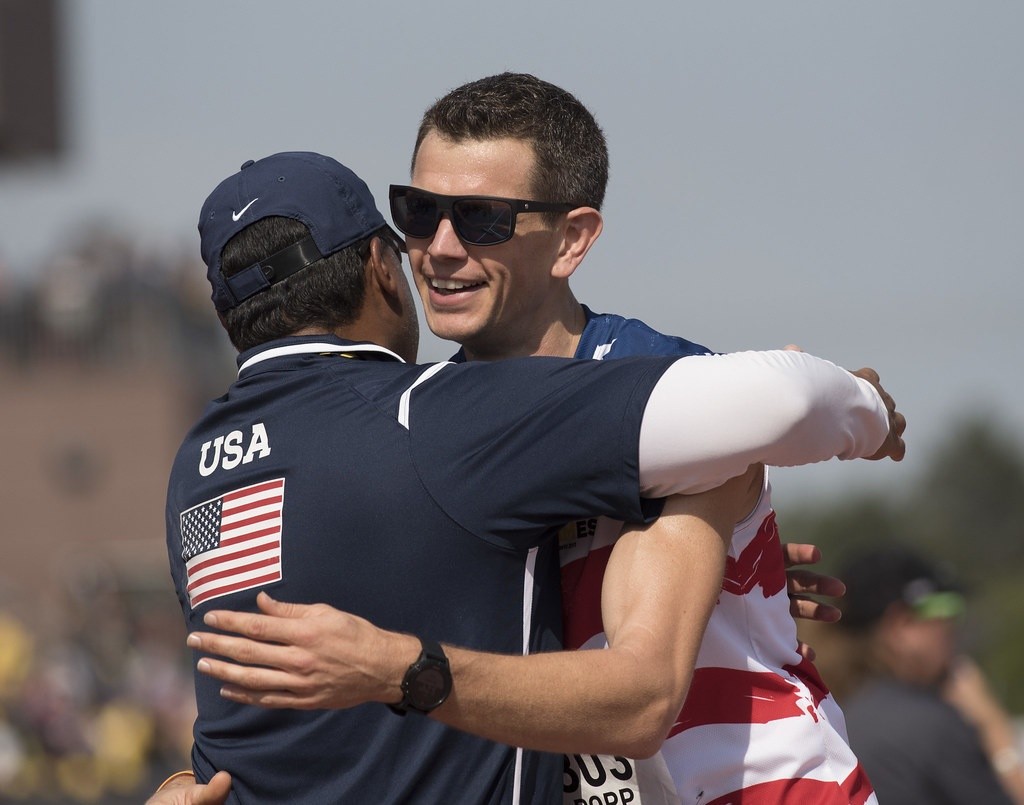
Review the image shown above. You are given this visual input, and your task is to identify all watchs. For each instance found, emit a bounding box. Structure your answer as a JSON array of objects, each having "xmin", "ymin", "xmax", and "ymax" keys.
[{"xmin": 387, "ymin": 631, "xmax": 453, "ymax": 717}]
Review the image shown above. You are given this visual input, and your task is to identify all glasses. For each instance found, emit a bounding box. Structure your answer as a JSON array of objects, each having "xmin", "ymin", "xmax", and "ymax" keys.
[{"xmin": 388, "ymin": 184, "xmax": 578, "ymax": 246}]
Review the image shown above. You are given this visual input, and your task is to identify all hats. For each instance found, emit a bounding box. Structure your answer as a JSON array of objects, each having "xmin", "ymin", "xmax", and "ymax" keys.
[{"xmin": 198, "ymin": 151, "xmax": 408, "ymax": 314}]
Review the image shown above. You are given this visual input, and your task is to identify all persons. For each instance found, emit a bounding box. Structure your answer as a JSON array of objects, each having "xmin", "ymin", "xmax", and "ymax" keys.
[
  {"xmin": 0, "ymin": 551, "xmax": 1023, "ymax": 801},
  {"xmin": 159, "ymin": 68, "xmax": 912, "ymax": 804}
]
